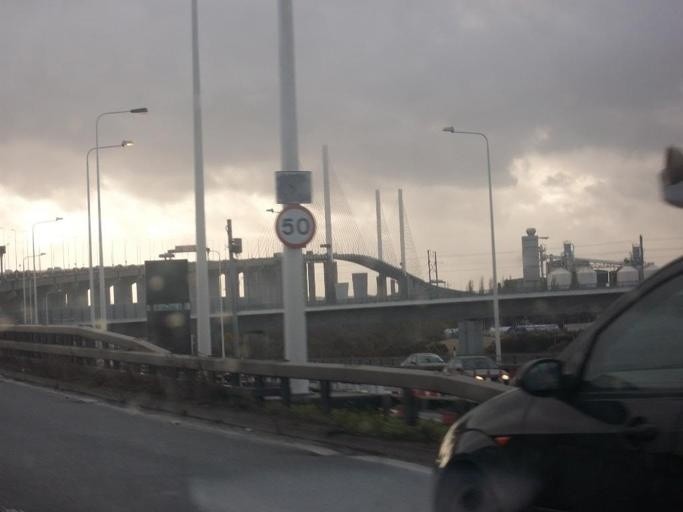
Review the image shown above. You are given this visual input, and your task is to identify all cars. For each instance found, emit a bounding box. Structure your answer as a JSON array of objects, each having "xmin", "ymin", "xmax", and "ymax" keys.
[
  {"xmin": 432, "ymin": 257, "xmax": 683, "ymax": 512},
  {"xmin": 401, "ymin": 352, "xmax": 509, "ymax": 386}
]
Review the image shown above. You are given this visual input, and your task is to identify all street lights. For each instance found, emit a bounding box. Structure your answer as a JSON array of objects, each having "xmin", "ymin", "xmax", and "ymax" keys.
[
  {"xmin": 83, "ymin": 93, "xmax": 149, "ymax": 360},
  {"xmin": 442, "ymin": 125, "xmax": 505, "ymax": 372},
  {"xmin": 22, "ymin": 216, "xmax": 64, "ymax": 324}
]
